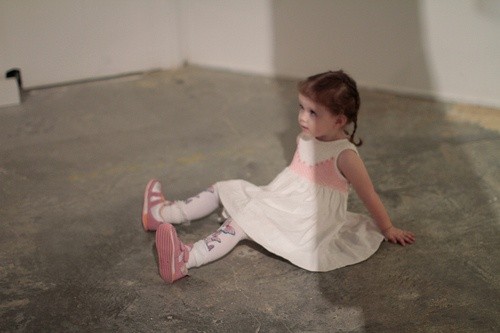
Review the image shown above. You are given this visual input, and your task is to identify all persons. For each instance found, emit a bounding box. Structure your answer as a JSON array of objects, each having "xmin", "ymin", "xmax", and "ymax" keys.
[{"xmin": 142, "ymin": 71, "xmax": 417, "ymax": 283}]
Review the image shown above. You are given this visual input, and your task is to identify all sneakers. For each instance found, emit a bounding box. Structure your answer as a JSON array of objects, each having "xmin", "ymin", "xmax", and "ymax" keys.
[
  {"xmin": 142, "ymin": 177, "xmax": 173, "ymax": 231},
  {"xmin": 156, "ymin": 223, "xmax": 193, "ymax": 282}
]
